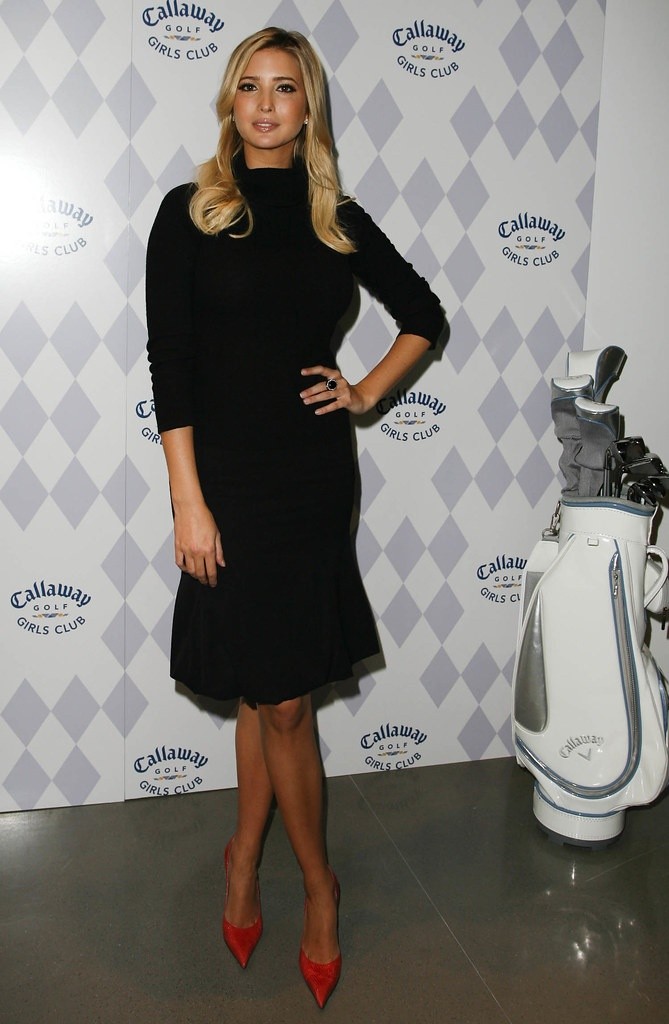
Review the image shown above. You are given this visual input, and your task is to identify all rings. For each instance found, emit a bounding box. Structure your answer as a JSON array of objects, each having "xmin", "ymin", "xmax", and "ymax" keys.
[{"xmin": 326, "ymin": 379, "xmax": 337, "ymax": 391}]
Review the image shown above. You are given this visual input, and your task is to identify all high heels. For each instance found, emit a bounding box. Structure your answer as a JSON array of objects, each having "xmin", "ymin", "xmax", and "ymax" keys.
[
  {"xmin": 223, "ymin": 833, "xmax": 263, "ymax": 968},
  {"xmin": 299, "ymin": 864, "xmax": 342, "ymax": 1009}
]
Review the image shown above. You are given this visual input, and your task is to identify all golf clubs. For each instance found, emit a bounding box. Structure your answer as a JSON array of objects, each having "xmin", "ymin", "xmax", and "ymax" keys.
[{"xmin": 603, "ymin": 437, "xmax": 669, "ymax": 510}]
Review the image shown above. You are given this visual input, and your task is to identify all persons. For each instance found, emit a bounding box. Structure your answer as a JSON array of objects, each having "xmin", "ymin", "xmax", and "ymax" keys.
[{"xmin": 146, "ymin": 26, "xmax": 444, "ymax": 1010}]
